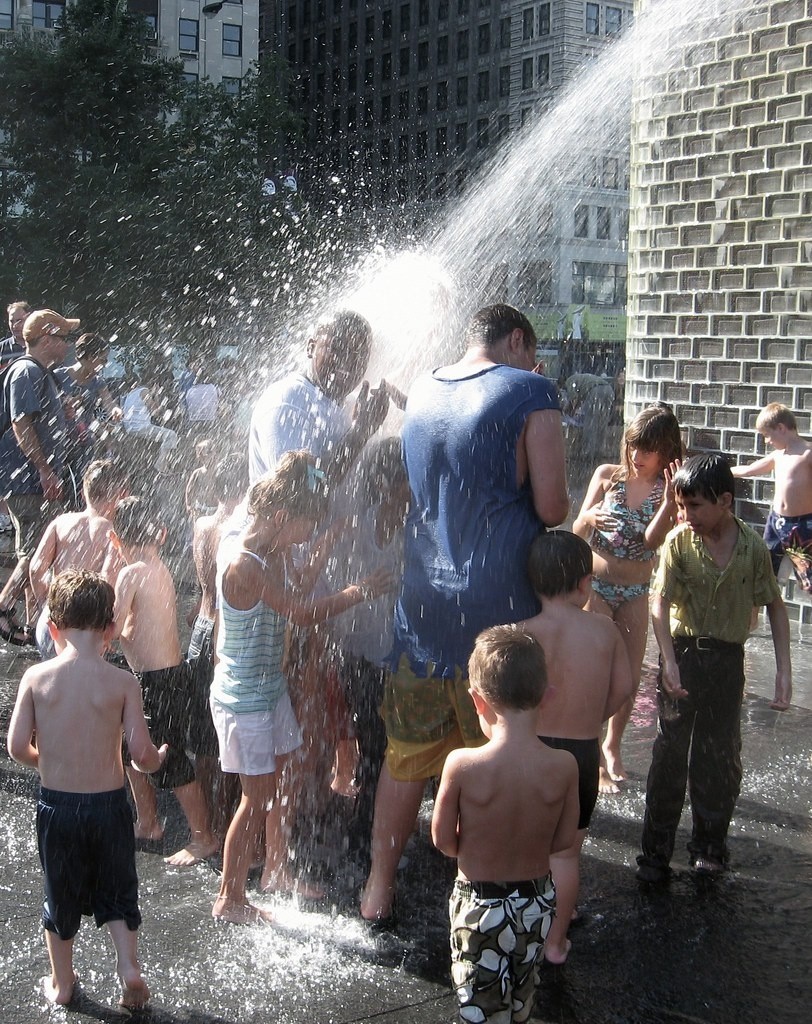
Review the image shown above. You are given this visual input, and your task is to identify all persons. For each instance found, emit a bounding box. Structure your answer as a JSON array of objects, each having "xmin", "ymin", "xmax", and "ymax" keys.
[
  {"xmin": 357, "ymin": 304, "xmax": 569, "ymax": 937},
  {"xmin": 2, "ymin": 300, "xmax": 412, "ymax": 926},
  {"xmin": 512, "ymin": 528, "xmax": 632, "ymax": 965},
  {"xmin": 631, "ymin": 451, "xmax": 793, "ymax": 904},
  {"xmin": 571, "ymin": 357, "xmax": 690, "ymax": 798},
  {"xmin": 6, "ymin": 574, "xmax": 170, "ymax": 1008},
  {"xmin": 731, "ymin": 402, "xmax": 812, "ymax": 630},
  {"xmin": 430, "ymin": 623, "xmax": 582, "ymax": 1024}
]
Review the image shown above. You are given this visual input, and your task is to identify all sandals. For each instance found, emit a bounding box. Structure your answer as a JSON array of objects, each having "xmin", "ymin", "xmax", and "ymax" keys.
[{"xmin": 0, "ymin": 607, "xmax": 39, "ymax": 645}]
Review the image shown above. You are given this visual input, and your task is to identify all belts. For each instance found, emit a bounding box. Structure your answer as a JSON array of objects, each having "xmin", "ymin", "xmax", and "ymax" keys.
[{"xmin": 678, "ymin": 636, "xmax": 741, "ymax": 654}]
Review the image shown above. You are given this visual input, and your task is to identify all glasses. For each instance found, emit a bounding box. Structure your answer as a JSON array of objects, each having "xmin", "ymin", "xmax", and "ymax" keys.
[{"xmin": 49, "ymin": 334, "xmax": 68, "ymax": 342}]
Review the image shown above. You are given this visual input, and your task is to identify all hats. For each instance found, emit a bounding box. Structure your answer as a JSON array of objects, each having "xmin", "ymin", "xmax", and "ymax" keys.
[{"xmin": 23, "ymin": 309, "xmax": 81, "ymax": 340}]
